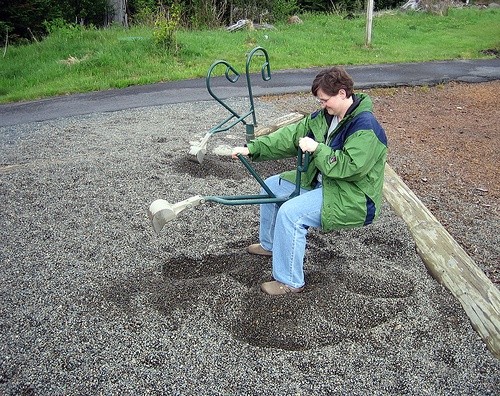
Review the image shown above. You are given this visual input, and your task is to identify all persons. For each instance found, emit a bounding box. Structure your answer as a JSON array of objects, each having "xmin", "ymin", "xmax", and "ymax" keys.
[{"xmin": 231, "ymin": 66, "xmax": 388, "ymax": 295}]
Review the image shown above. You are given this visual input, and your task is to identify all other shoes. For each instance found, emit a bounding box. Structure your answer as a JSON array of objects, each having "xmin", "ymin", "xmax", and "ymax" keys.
[
  {"xmin": 260, "ymin": 280, "xmax": 306, "ymax": 296},
  {"xmin": 247, "ymin": 244, "xmax": 272, "ymax": 256}
]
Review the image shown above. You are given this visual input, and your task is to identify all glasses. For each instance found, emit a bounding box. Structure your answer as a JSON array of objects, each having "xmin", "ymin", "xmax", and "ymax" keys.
[{"xmin": 318, "ymin": 95, "xmax": 333, "ymax": 105}]
